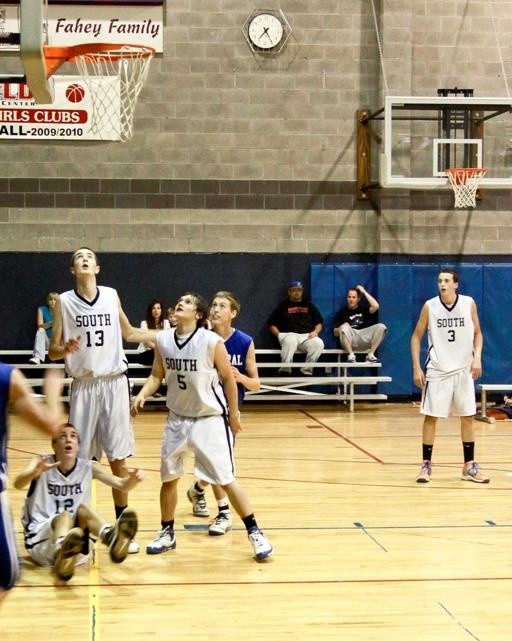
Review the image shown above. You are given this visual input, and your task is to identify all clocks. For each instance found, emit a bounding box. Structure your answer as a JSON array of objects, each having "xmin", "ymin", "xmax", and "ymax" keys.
[{"xmin": 247, "ymin": 12, "xmax": 284, "ymax": 49}]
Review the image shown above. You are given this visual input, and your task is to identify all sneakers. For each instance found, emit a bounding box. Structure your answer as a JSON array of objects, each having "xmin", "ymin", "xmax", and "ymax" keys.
[
  {"xmin": 54, "ymin": 527, "xmax": 85, "ymax": 581},
  {"xmin": 105, "ymin": 507, "xmax": 139, "ymax": 561},
  {"xmin": 417, "ymin": 460, "xmax": 432, "ymax": 482},
  {"xmin": 462, "ymin": 460, "xmax": 489, "ymax": 483},
  {"xmin": 30, "ymin": 357, "xmax": 40, "ymax": 364},
  {"xmin": 208, "ymin": 512, "xmax": 232, "ymax": 534},
  {"xmin": 248, "ymin": 526, "xmax": 272, "ymax": 559},
  {"xmin": 188, "ymin": 486, "xmax": 210, "ymax": 516},
  {"xmin": 366, "ymin": 355, "xmax": 377, "ymax": 362},
  {"xmin": 348, "ymin": 355, "xmax": 355, "ymax": 362},
  {"xmin": 147, "ymin": 531, "xmax": 176, "ymax": 554}
]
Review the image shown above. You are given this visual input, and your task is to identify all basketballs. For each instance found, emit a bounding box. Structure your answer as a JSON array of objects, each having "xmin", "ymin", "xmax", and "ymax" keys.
[{"xmin": 66, "ymin": 83, "xmax": 84, "ymax": 102}]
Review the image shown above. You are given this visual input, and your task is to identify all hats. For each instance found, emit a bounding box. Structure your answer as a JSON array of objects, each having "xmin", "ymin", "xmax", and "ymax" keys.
[{"xmin": 288, "ymin": 281, "xmax": 303, "ymax": 288}]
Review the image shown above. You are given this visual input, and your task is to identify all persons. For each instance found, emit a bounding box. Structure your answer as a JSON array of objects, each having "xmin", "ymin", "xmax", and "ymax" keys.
[
  {"xmin": 268, "ymin": 281, "xmax": 325, "ymax": 377},
  {"xmin": 167, "ymin": 307, "xmax": 179, "ymax": 327},
  {"xmin": 333, "ymin": 283, "xmax": 388, "ymax": 363},
  {"xmin": 29, "ymin": 291, "xmax": 58, "ymax": 366},
  {"xmin": 409, "ymin": 270, "xmax": 490, "ymax": 483},
  {"xmin": 0, "ymin": 363, "xmax": 65, "ymax": 590},
  {"xmin": 48, "ymin": 246, "xmax": 162, "ymax": 566},
  {"xmin": 136, "ymin": 298, "xmax": 172, "ymax": 398},
  {"xmin": 187, "ymin": 291, "xmax": 261, "ymax": 535},
  {"xmin": 130, "ymin": 291, "xmax": 274, "ymax": 561},
  {"xmin": 15, "ymin": 423, "xmax": 141, "ymax": 582}
]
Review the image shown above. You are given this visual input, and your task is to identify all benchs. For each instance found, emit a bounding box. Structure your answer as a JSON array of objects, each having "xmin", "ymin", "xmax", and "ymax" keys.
[
  {"xmin": 477, "ymin": 382, "xmax": 512, "ymax": 423},
  {"xmin": 243, "ymin": 349, "xmax": 392, "ymax": 411},
  {"xmin": 1, "ymin": 350, "xmax": 74, "ymax": 402},
  {"xmin": 124, "ymin": 349, "xmax": 167, "ymax": 401}
]
